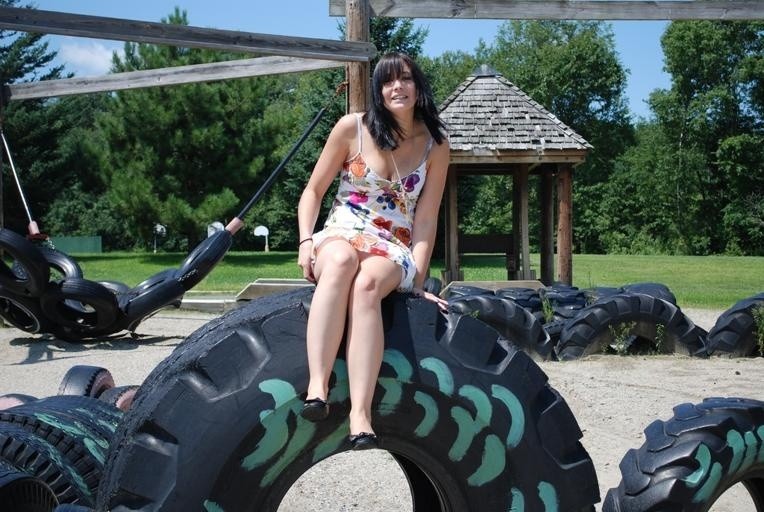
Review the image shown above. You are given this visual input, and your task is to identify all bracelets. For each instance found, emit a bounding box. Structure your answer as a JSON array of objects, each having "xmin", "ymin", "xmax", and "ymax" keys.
[{"xmin": 298, "ymin": 237, "xmax": 313, "ymax": 247}]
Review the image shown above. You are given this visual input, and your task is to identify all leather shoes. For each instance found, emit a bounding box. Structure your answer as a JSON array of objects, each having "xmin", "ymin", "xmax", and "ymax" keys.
[
  {"xmin": 348, "ymin": 430, "xmax": 380, "ymax": 450},
  {"xmin": 301, "ymin": 386, "xmax": 331, "ymax": 422}
]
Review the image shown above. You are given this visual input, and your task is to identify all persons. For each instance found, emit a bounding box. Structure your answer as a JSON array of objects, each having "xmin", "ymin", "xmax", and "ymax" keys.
[{"xmin": 294, "ymin": 49, "xmax": 452, "ymax": 452}]
